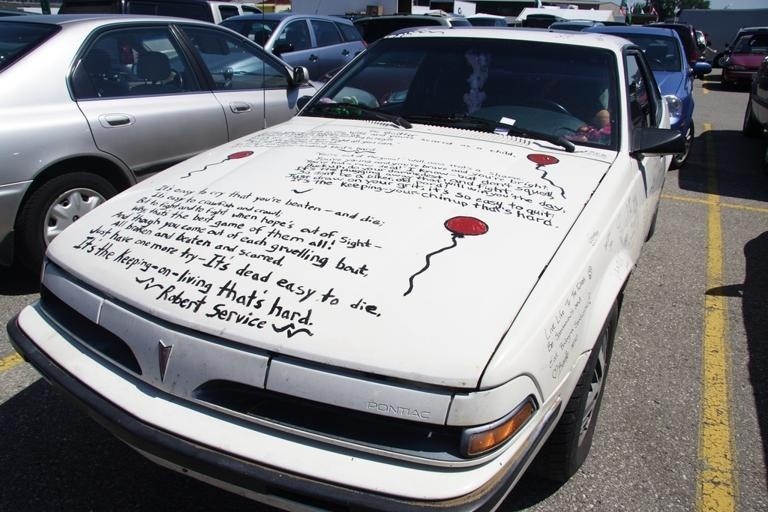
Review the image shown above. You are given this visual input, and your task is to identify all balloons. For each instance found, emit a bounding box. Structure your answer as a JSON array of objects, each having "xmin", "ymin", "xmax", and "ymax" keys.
[
  {"xmin": 443, "ymin": 217, "xmax": 489, "ymax": 235},
  {"xmin": 528, "ymin": 154, "xmax": 559, "ymax": 169},
  {"xmin": 226, "ymin": 152, "xmax": 251, "ymax": 160}
]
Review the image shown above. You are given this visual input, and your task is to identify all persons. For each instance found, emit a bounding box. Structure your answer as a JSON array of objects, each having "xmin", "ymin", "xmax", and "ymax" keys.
[{"xmin": 543, "ymin": 95, "xmax": 610, "ymax": 130}]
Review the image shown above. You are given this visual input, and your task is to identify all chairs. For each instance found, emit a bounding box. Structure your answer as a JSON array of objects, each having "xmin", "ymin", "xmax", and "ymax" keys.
[{"xmin": 130, "ymin": 52, "xmax": 178, "ymax": 92}]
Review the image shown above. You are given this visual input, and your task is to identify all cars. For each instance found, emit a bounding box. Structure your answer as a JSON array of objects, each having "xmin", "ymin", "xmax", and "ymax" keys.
[
  {"xmin": 721, "ymin": 31, "xmax": 768, "ymax": 91},
  {"xmin": 723, "ymin": 26, "xmax": 768, "ymax": 59},
  {"xmin": 743, "ymin": 54, "xmax": 768, "ymax": 174},
  {"xmin": 55, "ymin": 0, "xmax": 722, "ymax": 167},
  {"xmin": 3, "ymin": 22, "xmax": 689, "ymax": 512},
  {"xmin": 0, "ymin": 6, "xmax": 388, "ymax": 276}
]
